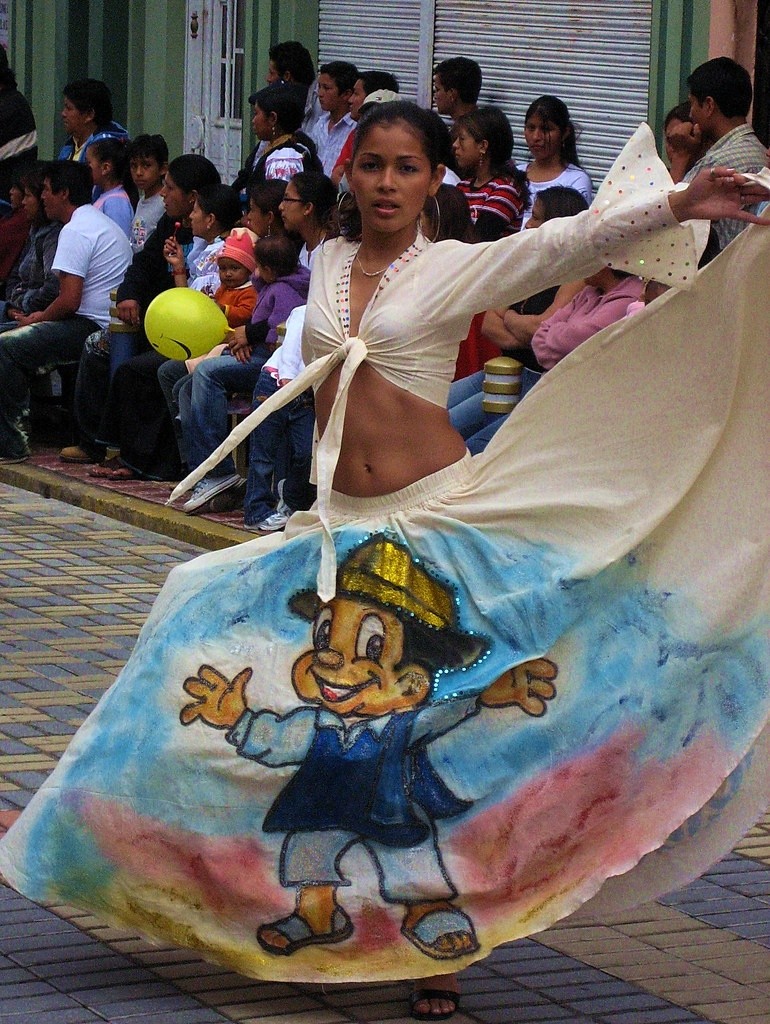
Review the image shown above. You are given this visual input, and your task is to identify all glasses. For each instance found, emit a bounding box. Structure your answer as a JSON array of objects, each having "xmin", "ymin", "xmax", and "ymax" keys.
[{"xmin": 282, "ymin": 197, "xmax": 306, "ymax": 206}]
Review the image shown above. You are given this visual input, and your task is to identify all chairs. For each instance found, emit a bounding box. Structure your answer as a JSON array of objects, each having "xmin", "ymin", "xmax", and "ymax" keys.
[{"xmin": 228, "ymin": 392, "xmax": 253, "ymax": 479}]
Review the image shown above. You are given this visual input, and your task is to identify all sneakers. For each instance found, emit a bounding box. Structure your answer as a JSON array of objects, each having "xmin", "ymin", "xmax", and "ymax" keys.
[
  {"xmin": 182, "ymin": 474, "xmax": 247, "ymax": 513},
  {"xmin": 60, "ymin": 445, "xmax": 92, "ymax": 463},
  {"xmin": 275, "ymin": 480, "xmax": 295, "ymax": 517},
  {"xmin": 244, "ymin": 511, "xmax": 288, "ymax": 532}
]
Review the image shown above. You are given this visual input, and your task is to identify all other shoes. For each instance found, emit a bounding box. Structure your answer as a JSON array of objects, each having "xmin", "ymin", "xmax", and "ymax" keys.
[{"xmin": 88, "ymin": 456, "xmax": 131, "ymax": 480}]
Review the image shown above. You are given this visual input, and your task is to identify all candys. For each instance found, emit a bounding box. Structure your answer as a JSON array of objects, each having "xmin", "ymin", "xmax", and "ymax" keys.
[{"xmin": 174, "ymin": 222, "xmax": 181, "ymax": 227}]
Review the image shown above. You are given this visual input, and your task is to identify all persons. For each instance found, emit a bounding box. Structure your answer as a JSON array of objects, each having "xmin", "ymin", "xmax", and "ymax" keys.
[
  {"xmin": 0, "ymin": 42, "xmax": 770, "ymax": 533},
  {"xmin": 298, "ymin": 100, "xmax": 770, "ymax": 1018}
]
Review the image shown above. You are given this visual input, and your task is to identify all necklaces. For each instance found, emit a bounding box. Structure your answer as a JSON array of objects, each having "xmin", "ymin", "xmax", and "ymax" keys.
[{"xmin": 356, "ymin": 255, "xmax": 389, "ymax": 277}]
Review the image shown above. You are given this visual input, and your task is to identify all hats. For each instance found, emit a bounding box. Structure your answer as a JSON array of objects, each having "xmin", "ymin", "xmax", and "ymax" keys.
[
  {"xmin": 216, "ymin": 227, "xmax": 262, "ymax": 270},
  {"xmin": 356, "ymin": 88, "xmax": 404, "ymax": 111}
]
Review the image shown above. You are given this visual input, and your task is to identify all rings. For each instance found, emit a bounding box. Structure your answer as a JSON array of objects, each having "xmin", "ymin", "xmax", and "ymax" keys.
[{"xmin": 711, "ymin": 168, "xmax": 717, "ymax": 180}]
